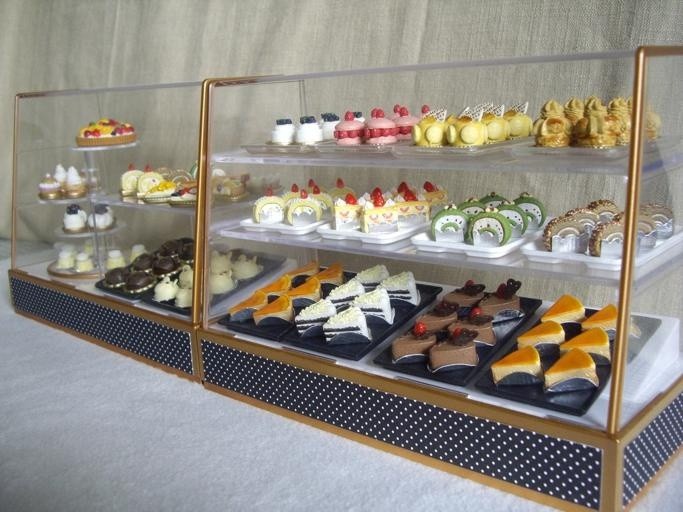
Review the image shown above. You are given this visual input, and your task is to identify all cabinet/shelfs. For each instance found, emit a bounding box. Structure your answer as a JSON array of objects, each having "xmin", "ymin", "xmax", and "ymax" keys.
[
  {"xmin": 7, "ymin": 75, "xmax": 309, "ymax": 384},
  {"xmin": 199, "ymin": 49, "xmax": 682, "ymax": 511}
]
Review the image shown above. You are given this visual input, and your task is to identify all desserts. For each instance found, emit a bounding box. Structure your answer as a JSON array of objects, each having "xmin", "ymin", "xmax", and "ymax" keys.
[{"xmin": 38, "ymin": 96, "xmax": 674, "ymax": 394}]
